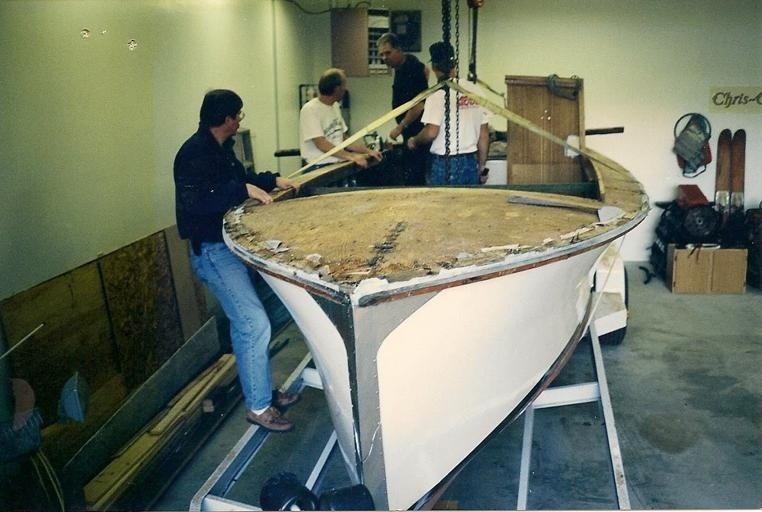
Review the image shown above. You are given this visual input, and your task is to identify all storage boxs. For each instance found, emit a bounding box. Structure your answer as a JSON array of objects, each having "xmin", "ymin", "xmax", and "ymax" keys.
[{"xmin": 667, "ymin": 246, "xmax": 748, "ymax": 294}]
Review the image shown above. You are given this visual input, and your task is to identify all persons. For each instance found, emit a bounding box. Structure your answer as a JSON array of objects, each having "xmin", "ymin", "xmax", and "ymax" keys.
[
  {"xmin": 407, "ymin": 41, "xmax": 489, "ymax": 187},
  {"xmin": 173, "ymin": 88, "xmax": 300, "ymax": 433},
  {"xmin": 377, "ymin": 33, "xmax": 429, "ymax": 187},
  {"xmin": 297, "ymin": 69, "xmax": 384, "ymax": 175}
]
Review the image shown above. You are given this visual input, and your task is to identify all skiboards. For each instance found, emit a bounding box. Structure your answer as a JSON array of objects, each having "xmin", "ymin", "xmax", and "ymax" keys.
[{"xmin": 714, "ymin": 128, "xmax": 746, "ymax": 215}]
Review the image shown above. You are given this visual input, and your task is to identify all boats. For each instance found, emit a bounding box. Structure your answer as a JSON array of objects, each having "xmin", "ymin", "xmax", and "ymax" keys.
[{"xmin": 221, "ymin": 119, "xmax": 651, "ymax": 512}]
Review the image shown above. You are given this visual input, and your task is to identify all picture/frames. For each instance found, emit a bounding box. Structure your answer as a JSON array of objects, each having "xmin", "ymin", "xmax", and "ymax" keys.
[{"xmin": 299, "ymin": 84, "xmax": 318, "ymax": 106}]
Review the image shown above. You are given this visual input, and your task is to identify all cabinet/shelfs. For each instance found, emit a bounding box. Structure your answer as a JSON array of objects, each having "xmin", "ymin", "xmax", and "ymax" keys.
[{"xmin": 332, "ymin": 8, "xmax": 393, "ymax": 80}]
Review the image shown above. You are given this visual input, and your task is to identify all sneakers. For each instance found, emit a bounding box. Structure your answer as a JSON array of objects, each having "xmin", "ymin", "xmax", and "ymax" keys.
[
  {"xmin": 245, "ymin": 406, "xmax": 295, "ymax": 433},
  {"xmin": 273, "ymin": 388, "xmax": 302, "ymax": 407}
]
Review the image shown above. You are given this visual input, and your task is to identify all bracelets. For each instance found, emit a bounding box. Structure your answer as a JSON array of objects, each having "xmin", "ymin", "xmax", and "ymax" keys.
[{"xmin": 401, "ymin": 120, "xmax": 408, "ymax": 129}]
